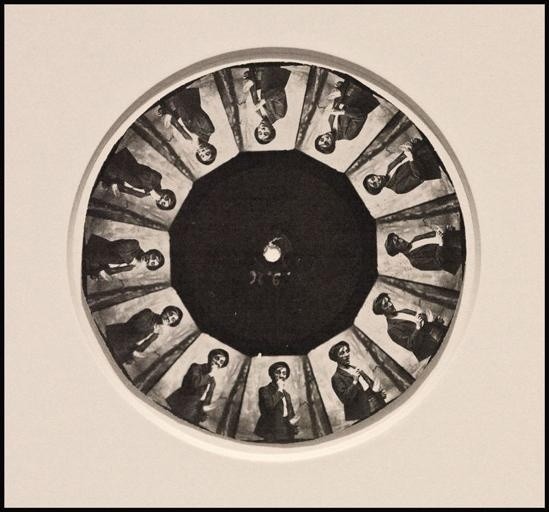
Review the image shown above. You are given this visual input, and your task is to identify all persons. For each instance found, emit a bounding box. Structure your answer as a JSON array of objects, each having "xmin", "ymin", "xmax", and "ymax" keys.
[{"xmin": 82, "ymin": 65, "xmax": 463, "ymax": 439}]
